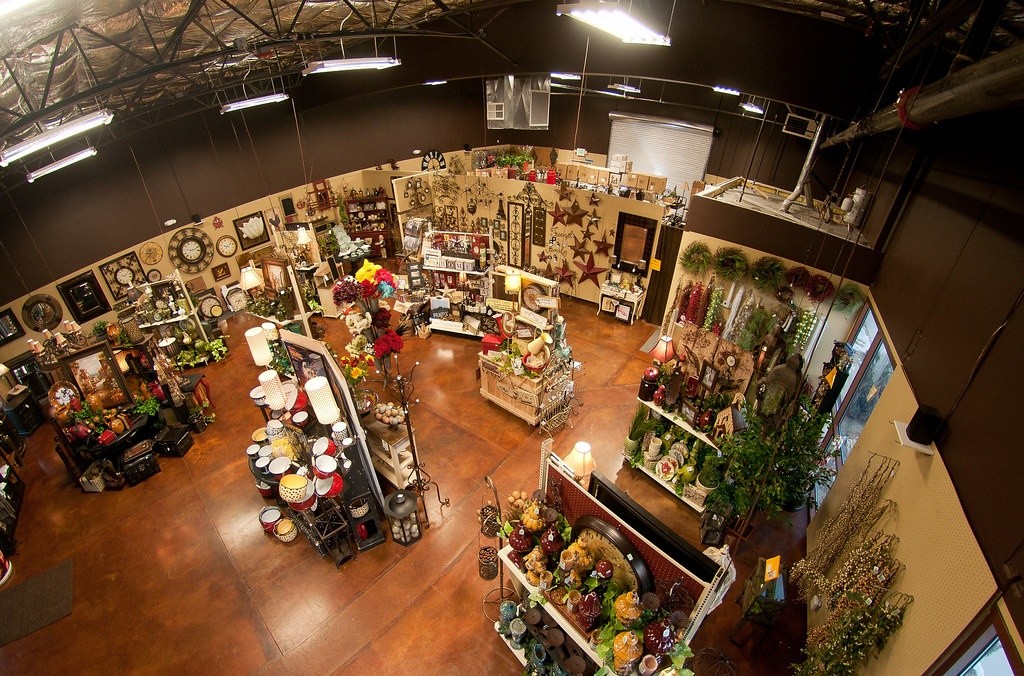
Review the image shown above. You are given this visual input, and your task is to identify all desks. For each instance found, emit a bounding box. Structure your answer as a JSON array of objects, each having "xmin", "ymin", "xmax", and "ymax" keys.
[
  {"xmin": 393, "ymin": 295, "xmax": 431, "ymax": 316},
  {"xmin": 294, "ymin": 262, "xmax": 318, "ymax": 290},
  {"xmin": 72, "ymin": 403, "xmax": 149, "ymax": 467},
  {"xmin": 215, "ymin": 309, "xmax": 245, "ymax": 324},
  {"xmin": 335, "ymin": 247, "xmax": 374, "ymax": 275},
  {"xmin": 597, "ymin": 290, "xmax": 643, "ymax": 325},
  {"xmin": 110, "ymin": 333, "xmax": 158, "ymax": 380},
  {"xmin": 178, "ymin": 374, "xmax": 217, "ymax": 416}
]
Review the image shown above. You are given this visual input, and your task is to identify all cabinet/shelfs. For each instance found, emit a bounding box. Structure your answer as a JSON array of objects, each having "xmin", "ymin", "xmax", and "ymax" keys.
[
  {"xmin": 619, "ymin": 397, "xmax": 726, "ymax": 515},
  {"xmin": 137, "ymin": 269, "xmax": 214, "ymax": 367},
  {"xmin": 665, "ymin": 194, "xmax": 687, "ymax": 228},
  {"xmin": 423, "ymin": 230, "xmax": 583, "ymax": 440},
  {"xmin": 3, "ymin": 392, "xmax": 46, "ymax": 436},
  {"xmin": 345, "ymin": 195, "xmax": 394, "ymax": 259},
  {"xmin": 0, "ymin": 444, "xmax": 25, "ymax": 556},
  {"xmin": 246, "ymin": 330, "xmax": 422, "ymax": 572},
  {"xmin": 246, "ymin": 261, "xmax": 356, "ymax": 380},
  {"xmin": 493, "ymin": 438, "xmax": 728, "ymax": 676}
]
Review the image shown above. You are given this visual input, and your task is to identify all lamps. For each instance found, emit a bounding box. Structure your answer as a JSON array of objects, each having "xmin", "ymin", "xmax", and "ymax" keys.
[
  {"xmin": 0, "ymin": 242, "xmax": 87, "ymax": 362},
  {"xmin": 127, "ymin": 286, "xmax": 147, "ymax": 325},
  {"xmin": 638, "ymin": 335, "xmax": 677, "ymax": 401},
  {"xmin": 243, "ymin": 322, "xmax": 353, "ymax": 543},
  {"xmin": 290, "ymin": 11, "xmax": 402, "ymax": 77},
  {"xmin": 0, "ymin": 50, "xmax": 115, "ymax": 183},
  {"xmin": 548, "ymin": 0, "xmax": 763, "ymax": 114},
  {"xmin": 159, "ymin": 336, "xmax": 190, "ymax": 386},
  {"xmin": 0, "ymin": 363, "xmax": 20, "ymax": 392},
  {"xmin": 240, "ymin": 266, "xmax": 264, "ymax": 301},
  {"xmin": 565, "ymin": 442, "xmax": 596, "ymax": 484},
  {"xmin": 207, "ymin": 72, "xmax": 289, "ymax": 114},
  {"xmin": 605, "ymin": 254, "xmax": 646, "ymax": 291},
  {"xmin": 505, "ymin": 269, "xmax": 522, "ymax": 317},
  {"xmin": 296, "ymin": 226, "xmax": 314, "ymax": 268}
]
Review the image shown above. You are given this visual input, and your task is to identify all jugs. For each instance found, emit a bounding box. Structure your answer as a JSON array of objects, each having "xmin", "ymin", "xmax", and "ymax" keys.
[{"xmin": 528, "ymin": 327, "xmax": 553, "ymax": 354}]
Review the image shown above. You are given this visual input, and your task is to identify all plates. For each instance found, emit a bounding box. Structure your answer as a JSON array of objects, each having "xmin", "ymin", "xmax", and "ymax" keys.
[{"xmin": 333, "ymin": 225, "xmax": 350, "ymax": 245}]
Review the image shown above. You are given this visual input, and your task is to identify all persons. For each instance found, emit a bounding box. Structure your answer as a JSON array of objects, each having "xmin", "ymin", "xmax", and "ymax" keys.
[{"xmin": 375, "ymin": 235, "xmax": 387, "ymax": 258}]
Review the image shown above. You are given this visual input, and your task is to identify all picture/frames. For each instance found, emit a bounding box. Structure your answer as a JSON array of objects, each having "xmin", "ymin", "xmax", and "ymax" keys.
[
  {"xmin": 678, "ymin": 398, "xmax": 700, "ymax": 429},
  {"xmin": 233, "ymin": 211, "xmax": 271, "ymax": 250},
  {"xmin": 600, "ymin": 293, "xmax": 632, "ymax": 324},
  {"xmin": 697, "ymin": 360, "xmax": 721, "ymax": 393},
  {"xmin": 211, "ymin": 262, "xmax": 231, "ymax": 282},
  {"xmin": 56, "ymin": 339, "xmax": 135, "ymax": 408},
  {"xmin": 266, "ymin": 261, "xmax": 287, "ymax": 293},
  {"xmin": 56, "ymin": 270, "xmax": 112, "ymax": 326}
]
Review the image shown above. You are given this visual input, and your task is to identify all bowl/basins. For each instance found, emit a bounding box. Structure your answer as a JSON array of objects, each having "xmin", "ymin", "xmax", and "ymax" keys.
[
  {"xmin": 651, "ymin": 437, "xmax": 662, "ymax": 450},
  {"xmin": 269, "ymin": 457, "xmax": 292, "ymax": 480},
  {"xmin": 259, "ymin": 507, "xmax": 297, "ymax": 543},
  {"xmin": 256, "ymin": 457, "xmax": 271, "ymax": 474},
  {"xmin": 247, "ymin": 444, "xmax": 260, "ymax": 459},
  {"xmin": 267, "ymin": 420, "xmax": 287, "ymax": 444},
  {"xmin": 250, "ymin": 386, "xmax": 266, "ymax": 405},
  {"xmin": 252, "ymin": 427, "xmax": 268, "ymax": 445},
  {"xmin": 313, "ymin": 437, "xmax": 336, "ymax": 457},
  {"xmin": 649, "ymin": 445, "xmax": 660, "ymax": 457},
  {"xmin": 259, "ymin": 445, "xmax": 274, "ymax": 460},
  {"xmin": 279, "ymin": 454, "xmax": 370, "ymax": 517},
  {"xmin": 293, "ymin": 411, "xmax": 308, "ymax": 427}
]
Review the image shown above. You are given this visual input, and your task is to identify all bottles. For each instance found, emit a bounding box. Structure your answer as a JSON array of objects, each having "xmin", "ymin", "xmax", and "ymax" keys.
[
  {"xmin": 372, "ymin": 188, "xmax": 378, "ymax": 196},
  {"xmin": 366, "ymin": 204, "xmax": 373, "ymax": 210},
  {"xmin": 359, "ymin": 188, "xmax": 363, "ymax": 197},
  {"xmin": 363, "ymin": 222, "xmax": 370, "ymax": 231},
  {"xmin": 379, "ymin": 184, "xmax": 384, "ymax": 196},
  {"xmin": 349, "ymin": 200, "xmax": 362, "ymax": 211},
  {"xmin": 344, "ymin": 187, "xmax": 347, "ymax": 192},
  {"xmin": 365, "ymin": 188, "xmax": 370, "ymax": 197},
  {"xmin": 350, "ymin": 188, "xmax": 358, "ymax": 199}
]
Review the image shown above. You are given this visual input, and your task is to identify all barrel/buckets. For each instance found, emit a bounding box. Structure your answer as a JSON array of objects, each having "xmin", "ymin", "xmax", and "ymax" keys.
[
  {"xmin": 289, "ymin": 392, "xmax": 308, "ymax": 416},
  {"xmin": 255, "ymin": 478, "xmax": 273, "ymax": 497}
]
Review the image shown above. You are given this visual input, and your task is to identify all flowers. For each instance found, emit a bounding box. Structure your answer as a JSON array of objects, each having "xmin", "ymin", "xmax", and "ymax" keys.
[{"xmin": 331, "ymin": 260, "xmax": 404, "ymax": 407}]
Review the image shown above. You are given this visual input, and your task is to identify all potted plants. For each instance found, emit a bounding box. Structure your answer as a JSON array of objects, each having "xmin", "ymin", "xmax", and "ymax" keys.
[
  {"xmin": 622, "ymin": 374, "xmax": 842, "ymax": 544},
  {"xmin": 204, "ymin": 333, "xmax": 231, "ymax": 365},
  {"xmin": 188, "ymin": 398, "xmax": 216, "ymax": 433}
]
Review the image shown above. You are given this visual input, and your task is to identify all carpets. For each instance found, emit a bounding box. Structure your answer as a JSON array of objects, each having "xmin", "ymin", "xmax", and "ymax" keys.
[{"xmin": 0, "ymin": 557, "xmax": 73, "ymax": 647}]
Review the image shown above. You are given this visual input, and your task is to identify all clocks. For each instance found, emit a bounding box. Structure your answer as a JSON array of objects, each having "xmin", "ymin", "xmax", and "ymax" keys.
[
  {"xmin": 97, "ymin": 241, "xmax": 164, "ymax": 301},
  {"xmin": 168, "ymin": 227, "xmax": 251, "ymax": 318},
  {"xmin": 571, "ymin": 515, "xmax": 651, "ymax": 601},
  {"xmin": 522, "ymin": 282, "xmax": 545, "ymax": 313}
]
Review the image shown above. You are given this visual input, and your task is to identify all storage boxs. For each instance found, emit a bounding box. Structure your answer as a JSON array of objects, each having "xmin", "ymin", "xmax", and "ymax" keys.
[
  {"xmin": 690, "ymin": 180, "xmax": 705, "ymax": 196},
  {"xmin": 481, "ymin": 333, "xmax": 504, "ymax": 355},
  {"xmin": 557, "ymin": 154, "xmax": 667, "ymax": 201}
]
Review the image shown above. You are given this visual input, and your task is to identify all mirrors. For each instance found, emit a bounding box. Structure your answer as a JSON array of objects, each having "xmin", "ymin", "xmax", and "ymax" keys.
[
  {"xmin": 0, "ymin": 307, "xmax": 26, "ymax": 347},
  {"xmin": 612, "ymin": 211, "xmax": 658, "ymax": 278}
]
